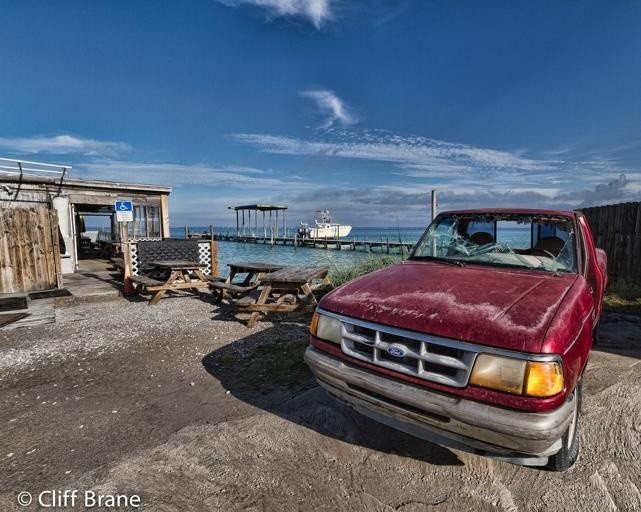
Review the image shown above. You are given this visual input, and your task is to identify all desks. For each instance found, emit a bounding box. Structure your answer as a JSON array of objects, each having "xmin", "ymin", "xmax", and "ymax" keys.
[{"xmin": 126, "ymin": 258, "xmax": 332, "ymax": 331}]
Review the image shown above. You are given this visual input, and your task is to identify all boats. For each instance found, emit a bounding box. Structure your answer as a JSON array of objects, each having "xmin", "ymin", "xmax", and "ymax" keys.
[{"xmin": 298, "ymin": 209, "xmax": 352, "ymax": 239}]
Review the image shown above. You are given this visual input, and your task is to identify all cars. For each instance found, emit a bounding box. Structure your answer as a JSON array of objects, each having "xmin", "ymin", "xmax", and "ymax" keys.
[{"xmin": 303, "ymin": 208, "xmax": 609, "ymax": 472}]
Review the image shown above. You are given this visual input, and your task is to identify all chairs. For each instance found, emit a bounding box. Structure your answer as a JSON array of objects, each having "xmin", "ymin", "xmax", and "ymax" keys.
[
  {"xmin": 464, "ymin": 232, "xmax": 495, "ymax": 253},
  {"xmin": 532, "ymin": 236, "xmax": 569, "ymax": 262}
]
